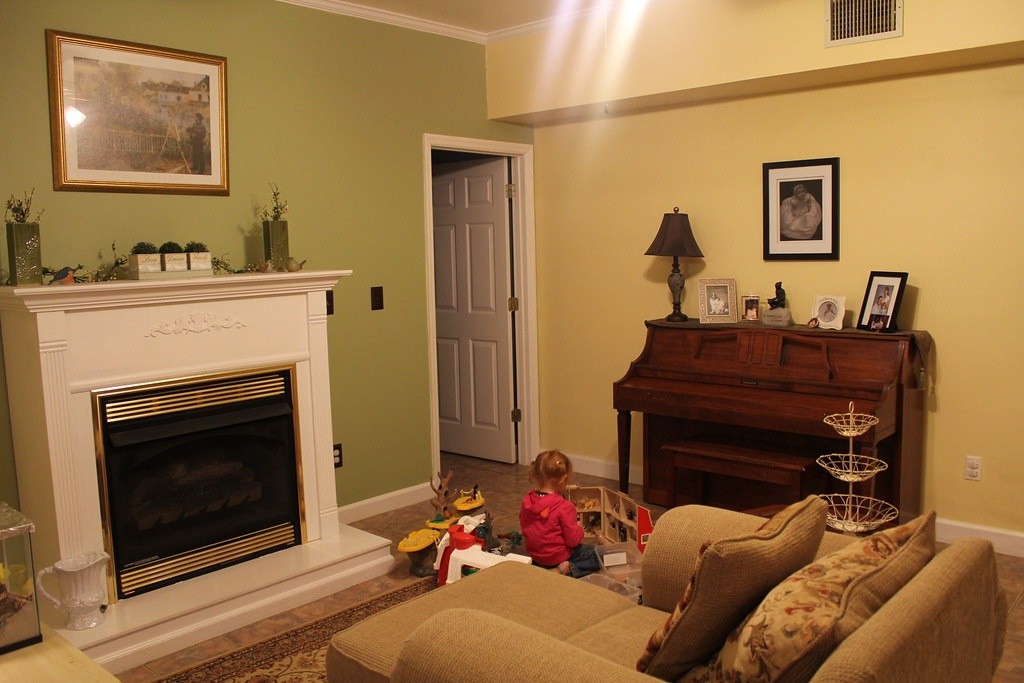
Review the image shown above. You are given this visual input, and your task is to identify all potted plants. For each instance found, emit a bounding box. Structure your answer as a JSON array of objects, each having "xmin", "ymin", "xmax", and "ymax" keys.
[
  {"xmin": 127, "ymin": 241, "xmax": 161, "ymax": 270},
  {"xmin": 260, "ymin": 180, "xmax": 292, "ymax": 272},
  {"xmin": 2, "ymin": 188, "xmax": 47, "ymax": 286},
  {"xmin": 186, "ymin": 240, "xmax": 216, "ymax": 270},
  {"xmin": 160, "ymin": 237, "xmax": 188, "ymax": 272}
]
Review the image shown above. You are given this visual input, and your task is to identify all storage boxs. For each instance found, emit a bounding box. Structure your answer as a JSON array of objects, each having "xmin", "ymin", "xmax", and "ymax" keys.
[
  {"xmin": 592, "ymin": 540, "xmax": 645, "ymax": 586},
  {"xmin": 0, "ymin": 499, "xmax": 46, "ymax": 652},
  {"xmin": 577, "ymin": 572, "xmax": 642, "ymax": 603}
]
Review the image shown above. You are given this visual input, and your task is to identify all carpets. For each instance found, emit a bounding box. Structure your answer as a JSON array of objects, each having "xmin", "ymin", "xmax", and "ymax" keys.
[{"xmin": 150, "ymin": 576, "xmax": 438, "ymax": 683}]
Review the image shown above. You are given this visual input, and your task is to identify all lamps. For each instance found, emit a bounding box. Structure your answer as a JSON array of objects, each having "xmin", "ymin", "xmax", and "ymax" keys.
[
  {"xmin": 638, "ymin": 206, "xmax": 703, "ymax": 322},
  {"xmin": 63, "ymin": 101, "xmax": 86, "ymax": 129}
]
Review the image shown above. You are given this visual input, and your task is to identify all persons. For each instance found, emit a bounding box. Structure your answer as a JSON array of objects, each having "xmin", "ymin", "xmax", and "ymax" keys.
[
  {"xmin": 743, "ymin": 303, "xmax": 758, "ymax": 320},
  {"xmin": 866, "ymin": 316, "xmax": 883, "ymax": 332},
  {"xmin": 710, "ymin": 292, "xmax": 729, "ymax": 315},
  {"xmin": 871, "ymin": 287, "xmax": 890, "ymax": 314},
  {"xmin": 824, "ymin": 304, "xmax": 835, "ymax": 321},
  {"xmin": 184, "ymin": 113, "xmax": 206, "ymax": 173},
  {"xmin": 767, "ymin": 282, "xmax": 785, "ymax": 310},
  {"xmin": 519, "ymin": 450, "xmax": 603, "ymax": 579},
  {"xmin": 789, "ymin": 184, "xmax": 813, "ymax": 234}
]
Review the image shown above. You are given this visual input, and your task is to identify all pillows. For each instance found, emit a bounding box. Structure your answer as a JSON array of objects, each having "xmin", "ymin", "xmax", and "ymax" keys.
[
  {"xmin": 634, "ymin": 494, "xmax": 835, "ymax": 672},
  {"xmin": 683, "ymin": 508, "xmax": 939, "ymax": 683}
]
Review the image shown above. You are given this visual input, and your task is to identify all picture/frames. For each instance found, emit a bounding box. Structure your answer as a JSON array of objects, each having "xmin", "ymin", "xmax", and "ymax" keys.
[
  {"xmin": 812, "ymin": 293, "xmax": 846, "ymax": 327},
  {"xmin": 43, "ymin": 27, "xmax": 230, "ymax": 197},
  {"xmin": 697, "ymin": 275, "xmax": 738, "ymax": 324},
  {"xmin": 856, "ymin": 269, "xmax": 910, "ymax": 330},
  {"xmin": 741, "ymin": 294, "xmax": 760, "ymax": 322},
  {"xmin": 761, "ymin": 156, "xmax": 841, "ymax": 262}
]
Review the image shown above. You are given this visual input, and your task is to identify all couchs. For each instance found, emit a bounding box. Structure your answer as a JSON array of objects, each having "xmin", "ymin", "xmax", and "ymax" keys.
[{"xmin": 323, "ymin": 502, "xmax": 1012, "ymax": 683}]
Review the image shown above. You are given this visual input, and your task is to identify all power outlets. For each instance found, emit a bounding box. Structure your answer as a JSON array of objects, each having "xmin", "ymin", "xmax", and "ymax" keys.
[
  {"xmin": 332, "ymin": 442, "xmax": 343, "ymax": 467},
  {"xmin": 963, "ymin": 456, "xmax": 985, "ymax": 481}
]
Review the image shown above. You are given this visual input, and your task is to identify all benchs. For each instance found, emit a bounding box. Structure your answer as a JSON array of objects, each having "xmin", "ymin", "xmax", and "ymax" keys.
[{"xmin": 660, "ymin": 432, "xmax": 824, "ymax": 511}]
